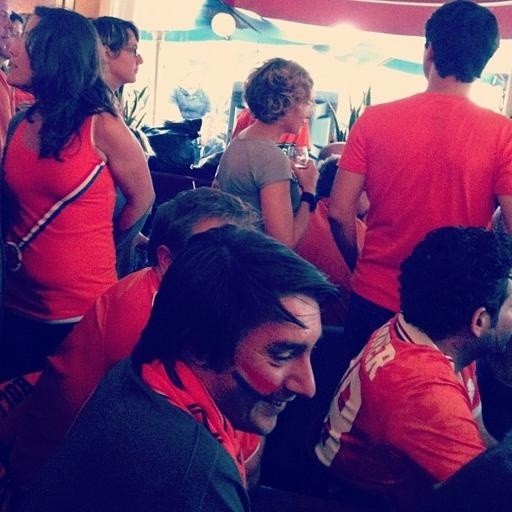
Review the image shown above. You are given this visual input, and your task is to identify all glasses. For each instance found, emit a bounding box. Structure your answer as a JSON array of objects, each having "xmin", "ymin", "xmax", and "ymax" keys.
[{"xmin": 115, "ymin": 47, "xmax": 138, "ymax": 57}]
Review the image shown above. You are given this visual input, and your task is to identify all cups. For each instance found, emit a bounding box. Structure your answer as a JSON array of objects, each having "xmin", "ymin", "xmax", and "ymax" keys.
[{"xmin": 288, "ymin": 146, "xmax": 309, "ymax": 169}]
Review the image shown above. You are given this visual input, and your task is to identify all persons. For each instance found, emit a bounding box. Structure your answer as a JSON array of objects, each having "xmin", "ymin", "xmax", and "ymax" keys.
[
  {"xmin": 1, "ymin": 2, "xmax": 156, "ymax": 356},
  {"xmin": 2, "ymin": 141, "xmax": 512, "ymax": 511},
  {"xmin": 168, "ymin": 64, "xmax": 212, "ymax": 121},
  {"xmin": 327, "ymin": 1, "xmax": 511, "ymax": 359},
  {"xmin": 210, "ymin": 56, "xmax": 320, "ymax": 247}
]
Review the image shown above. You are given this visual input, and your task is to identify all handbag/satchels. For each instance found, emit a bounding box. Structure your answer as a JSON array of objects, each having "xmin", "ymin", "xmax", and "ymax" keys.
[{"xmin": 137, "ymin": 128, "xmax": 200, "ymax": 174}]
